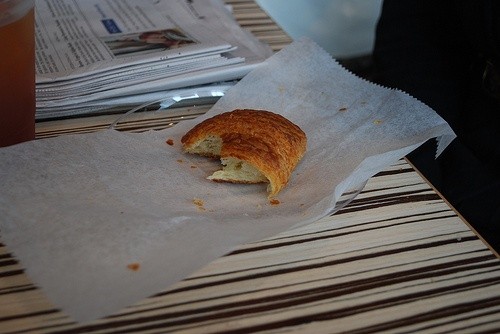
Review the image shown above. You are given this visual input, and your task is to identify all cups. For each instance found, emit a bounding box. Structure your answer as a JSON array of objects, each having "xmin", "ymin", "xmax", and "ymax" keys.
[{"xmin": 1, "ymin": 0, "xmax": 37, "ymax": 149}]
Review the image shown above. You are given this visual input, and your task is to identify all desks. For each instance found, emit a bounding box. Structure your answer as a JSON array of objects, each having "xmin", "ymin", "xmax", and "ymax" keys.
[{"xmin": 1, "ymin": 1, "xmax": 499, "ymax": 334}]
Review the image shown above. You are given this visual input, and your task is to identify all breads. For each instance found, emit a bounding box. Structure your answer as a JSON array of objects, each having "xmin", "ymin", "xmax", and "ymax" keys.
[{"xmin": 179, "ymin": 109, "xmax": 307, "ymax": 199}]
